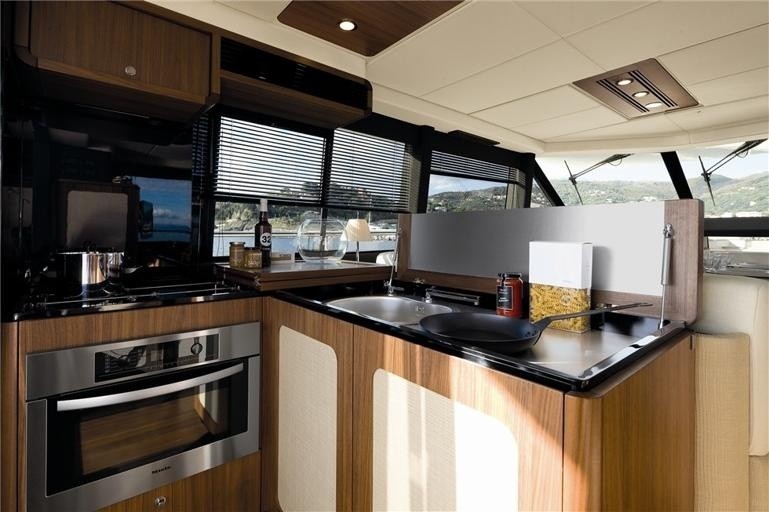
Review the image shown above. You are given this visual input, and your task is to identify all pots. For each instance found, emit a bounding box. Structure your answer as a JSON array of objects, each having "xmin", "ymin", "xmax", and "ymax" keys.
[
  {"xmin": 57, "ymin": 239, "xmax": 109, "ymax": 294},
  {"xmin": 419, "ymin": 301, "xmax": 655, "ymax": 358},
  {"xmin": 105, "ymin": 245, "xmax": 127, "ymax": 283}
]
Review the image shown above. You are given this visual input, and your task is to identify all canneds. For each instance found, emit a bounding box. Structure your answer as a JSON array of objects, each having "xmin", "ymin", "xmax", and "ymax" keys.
[
  {"xmin": 229, "ymin": 242, "xmax": 246, "ymax": 267},
  {"xmin": 495, "ymin": 272, "xmax": 524, "ymax": 319},
  {"xmin": 243, "ymin": 246, "xmax": 263, "ymax": 269}
]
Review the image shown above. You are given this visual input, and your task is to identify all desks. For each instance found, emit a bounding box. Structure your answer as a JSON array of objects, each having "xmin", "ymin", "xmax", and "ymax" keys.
[{"xmin": 211, "ymin": 259, "xmax": 396, "ymax": 300}]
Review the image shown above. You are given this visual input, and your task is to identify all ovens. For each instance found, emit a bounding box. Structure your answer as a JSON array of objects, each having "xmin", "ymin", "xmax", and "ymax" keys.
[{"xmin": 25, "ymin": 320, "xmax": 262, "ymax": 512}]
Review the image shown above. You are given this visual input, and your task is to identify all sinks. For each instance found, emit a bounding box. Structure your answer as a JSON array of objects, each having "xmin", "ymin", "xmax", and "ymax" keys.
[{"xmin": 326, "ymin": 296, "xmax": 452, "ymax": 328}]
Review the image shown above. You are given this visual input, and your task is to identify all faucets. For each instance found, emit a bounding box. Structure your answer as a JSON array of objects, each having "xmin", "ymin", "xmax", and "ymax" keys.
[{"xmin": 425, "ymin": 288, "xmax": 480, "ymax": 307}]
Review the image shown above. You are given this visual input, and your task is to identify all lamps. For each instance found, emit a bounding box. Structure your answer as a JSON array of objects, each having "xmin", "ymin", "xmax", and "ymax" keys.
[{"xmin": 339, "ymin": 216, "xmax": 373, "ymax": 261}]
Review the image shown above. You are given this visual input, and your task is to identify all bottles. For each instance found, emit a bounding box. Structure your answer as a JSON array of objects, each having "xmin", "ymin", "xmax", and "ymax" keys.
[{"xmin": 255, "ymin": 199, "xmax": 272, "ymax": 268}]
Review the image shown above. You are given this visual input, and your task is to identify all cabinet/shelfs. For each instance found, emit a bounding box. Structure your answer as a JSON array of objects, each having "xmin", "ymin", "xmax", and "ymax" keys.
[
  {"xmin": 258, "ymin": 296, "xmax": 696, "ymax": 511},
  {"xmin": 0, "ymin": 0, "xmax": 222, "ymax": 115},
  {"xmin": 211, "ymin": 22, "xmax": 372, "ymax": 129}
]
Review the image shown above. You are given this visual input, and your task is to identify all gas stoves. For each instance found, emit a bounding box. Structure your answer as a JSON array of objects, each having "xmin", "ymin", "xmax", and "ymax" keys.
[{"xmin": 14, "ymin": 264, "xmax": 242, "ymax": 320}]
